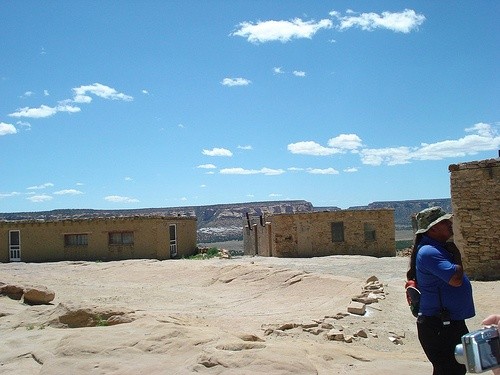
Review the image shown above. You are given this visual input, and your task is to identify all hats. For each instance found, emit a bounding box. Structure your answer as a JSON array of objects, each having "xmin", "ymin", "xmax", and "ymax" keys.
[{"xmin": 415, "ymin": 206, "xmax": 453, "ymax": 235}]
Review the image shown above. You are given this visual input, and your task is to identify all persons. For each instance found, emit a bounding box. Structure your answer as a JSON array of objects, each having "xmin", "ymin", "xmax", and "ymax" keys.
[
  {"xmin": 482, "ymin": 315, "xmax": 500, "ymax": 375},
  {"xmin": 407, "ymin": 206, "xmax": 476, "ymax": 375}
]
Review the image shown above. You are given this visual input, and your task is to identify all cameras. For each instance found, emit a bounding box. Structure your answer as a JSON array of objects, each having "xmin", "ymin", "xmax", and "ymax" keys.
[{"xmin": 455, "ymin": 325, "xmax": 500, "ymax": 373}]
